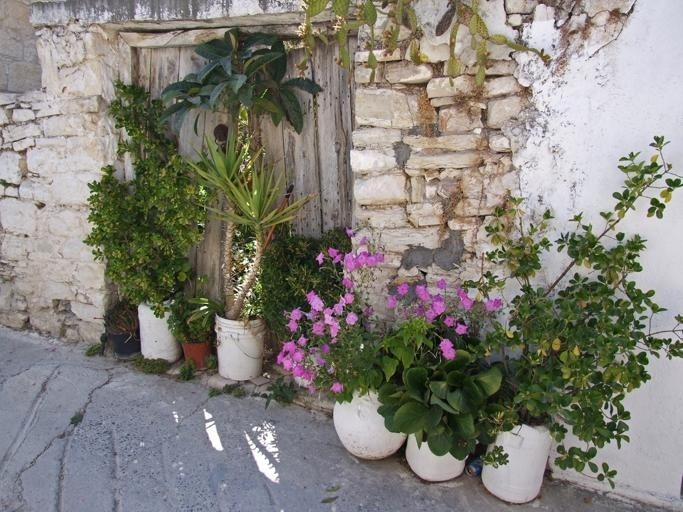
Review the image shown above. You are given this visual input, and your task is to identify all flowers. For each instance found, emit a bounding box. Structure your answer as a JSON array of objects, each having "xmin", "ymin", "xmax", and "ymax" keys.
[{"xmin": 275, "ymin": 227, "xmax": 503, "ymax": 402}]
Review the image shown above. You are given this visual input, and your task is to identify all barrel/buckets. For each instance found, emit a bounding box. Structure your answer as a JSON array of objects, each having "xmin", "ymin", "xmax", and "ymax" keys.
[
  {"xmin": 481, "ymin": 420, "xmax": 551, "ymax": 505},
  {"xmin": 215, "ymin": 309, "xmax": 265, "ymax": 381},
  {"xmin": 405, "ymin": 434, "xmax": 480, "ymax": 483},
  {"xmin": 138, "ymin": 297, "xmax": 183, "ymax": 363}
]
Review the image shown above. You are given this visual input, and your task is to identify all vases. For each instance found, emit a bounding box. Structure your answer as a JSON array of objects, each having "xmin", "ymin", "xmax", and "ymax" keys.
[{"xmin": 330, "ymin": 386, "xmax": 410, "ymax": 459}]
